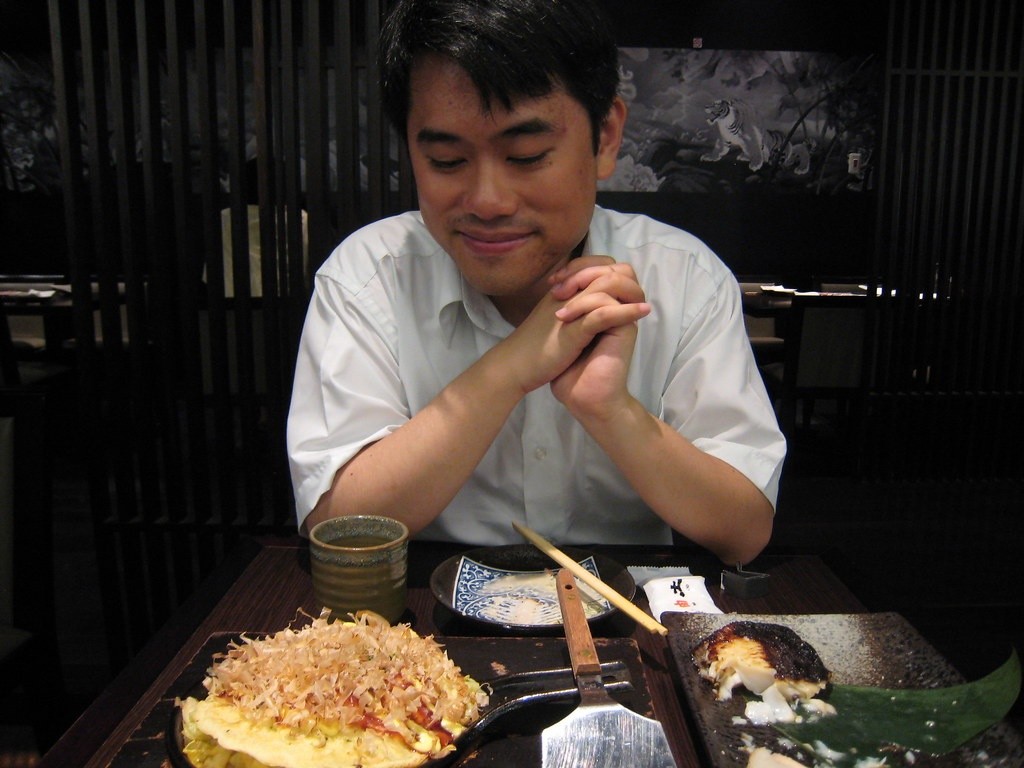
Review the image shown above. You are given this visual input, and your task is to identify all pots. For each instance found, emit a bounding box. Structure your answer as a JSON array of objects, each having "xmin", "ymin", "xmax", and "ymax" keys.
[{"xmin": 172, "ymin": 659, "xmax": 634, "ymax": 767}]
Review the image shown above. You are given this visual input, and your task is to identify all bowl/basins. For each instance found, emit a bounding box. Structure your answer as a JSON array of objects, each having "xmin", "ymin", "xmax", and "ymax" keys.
[{"xmin": 429, "ymin": 544, "xmax": 637, "ymax": 636}]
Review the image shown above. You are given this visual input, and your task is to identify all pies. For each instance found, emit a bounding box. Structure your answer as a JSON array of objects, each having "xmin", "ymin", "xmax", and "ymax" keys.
[{"xmin": 192, "ymin": 688, "xmax": 428, "ymax": 768}]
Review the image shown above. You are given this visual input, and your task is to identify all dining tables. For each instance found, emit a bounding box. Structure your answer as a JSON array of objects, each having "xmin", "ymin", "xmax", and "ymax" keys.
[{"xmin": 39, "ymin": 533, "xmax": 869, "ymax": 768}]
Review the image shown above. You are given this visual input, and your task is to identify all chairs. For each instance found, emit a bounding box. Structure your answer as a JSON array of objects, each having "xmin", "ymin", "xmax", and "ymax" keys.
[
  {"xmin": 0, "ymin": 301, "xmax": 84, "ymax": 750},
  {"xmin": 736, "ymin": 270, "xmax": 874, "ymax": 475}
]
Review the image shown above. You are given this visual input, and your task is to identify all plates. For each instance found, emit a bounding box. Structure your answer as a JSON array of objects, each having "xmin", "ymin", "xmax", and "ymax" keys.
[{"xmin": 660, "ymin": 609, "xmax": 1024, "ymax": 768}]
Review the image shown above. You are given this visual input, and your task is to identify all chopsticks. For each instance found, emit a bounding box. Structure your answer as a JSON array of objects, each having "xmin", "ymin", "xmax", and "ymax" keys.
[{"xmin": 511, "ymin": 520, "xmax": 668, "ymax": 636}]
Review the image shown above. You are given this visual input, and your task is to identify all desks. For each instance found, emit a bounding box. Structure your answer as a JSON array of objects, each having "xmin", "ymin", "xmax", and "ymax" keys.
[
  {"xmin": 743, "ymin": 291, "xmax": 949, "ymax": 319},
  {"xmin": 1, "ymin": 283, "xmax": 126, "ymax": 315}
]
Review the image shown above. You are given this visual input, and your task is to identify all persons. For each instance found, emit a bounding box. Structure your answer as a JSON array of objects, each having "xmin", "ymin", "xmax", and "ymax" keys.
[{"xmin": 284, "ymin": 0, "xmax": 789, "ymax": 564}]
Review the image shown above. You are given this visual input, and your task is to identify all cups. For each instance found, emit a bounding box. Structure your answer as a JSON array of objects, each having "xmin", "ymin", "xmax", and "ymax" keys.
[{"xmin": 309, "ymin": 515, "xmax": 409, "ymax": 624}]
